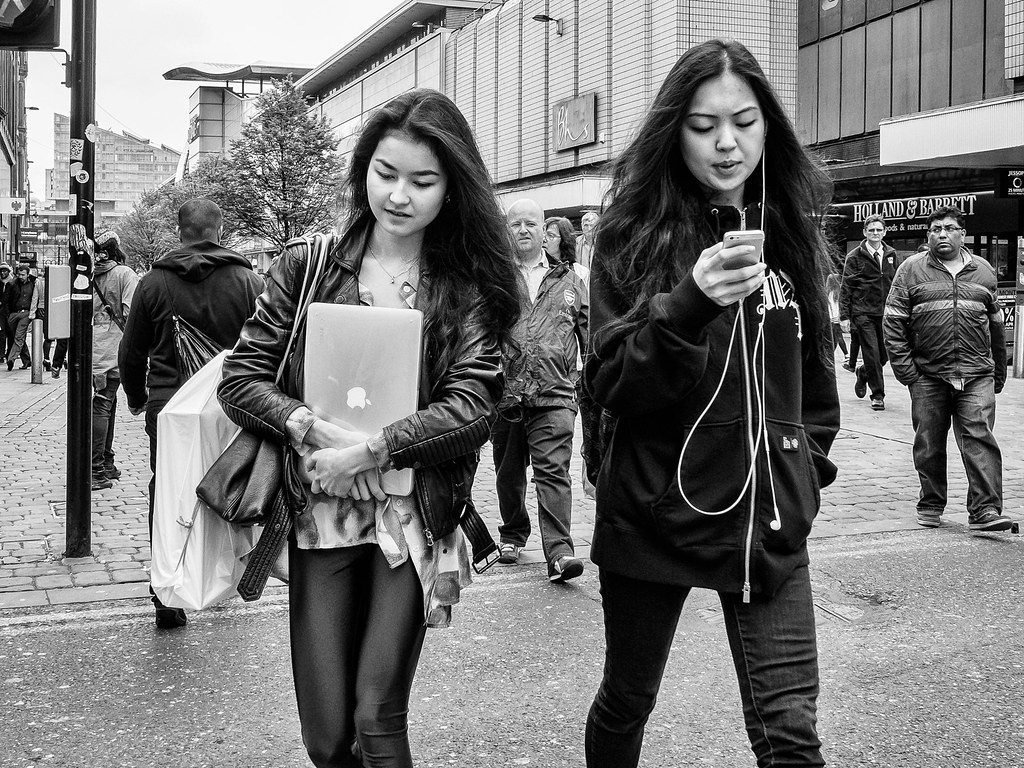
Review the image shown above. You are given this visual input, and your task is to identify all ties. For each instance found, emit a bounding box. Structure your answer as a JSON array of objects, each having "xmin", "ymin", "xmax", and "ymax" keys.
[{"xmin": 874, "ymin": 252, "xmax": 880, "ymax": 268}]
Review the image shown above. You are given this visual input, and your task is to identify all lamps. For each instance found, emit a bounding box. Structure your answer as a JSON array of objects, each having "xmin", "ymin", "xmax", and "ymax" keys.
[
  {"xmin": 28, "ymin": 209, "xmax": 38, "ymax": 218},
  {"xmin": 25, "ymin": 107, "xmax": 40, "ymax": 110},
  {"xmin": 412, "ymin": 20, "xmax": 437, "ymax": 30},
  {"xmin": 532, "ymin": 15, "xmax": 562, "ymax": 36}
]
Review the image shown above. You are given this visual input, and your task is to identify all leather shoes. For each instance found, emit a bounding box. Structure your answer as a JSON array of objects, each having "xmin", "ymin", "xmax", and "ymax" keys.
[
  {"xmin": 871, "ymin": 397, "xmax": 885, "ymax": 410},
  {"xmin": 6, "ymin": 360, "xmax": 13, "ymax": 371},
  {"xmin": 855, "ymin": 366, "xmax": 866, "ymax": 399},
  {"xmin": 19, "ymin": 361, "xmax": 32, "ymax": 369}
]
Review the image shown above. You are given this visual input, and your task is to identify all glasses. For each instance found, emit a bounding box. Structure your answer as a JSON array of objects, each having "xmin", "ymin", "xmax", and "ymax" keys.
[
  {"xmin": 549, "ymin": 233, "xmax": 561, "ymax": 241},
  {"xmin": 929, "ymin": 225, "xmax": 961, "ymax": 233}
]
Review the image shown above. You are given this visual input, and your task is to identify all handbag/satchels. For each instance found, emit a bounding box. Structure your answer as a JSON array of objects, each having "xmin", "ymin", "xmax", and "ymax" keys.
[
  {"xmin": 197, "ymin": 234, "xmax": 331, "ymax": 521},
  {"xmin": 170, "ymin": 309, "xmax": 221, "ymax": 383},
  {"xmin": 152, "ymin": 232, "xmax": 328, "ymax": 608}
]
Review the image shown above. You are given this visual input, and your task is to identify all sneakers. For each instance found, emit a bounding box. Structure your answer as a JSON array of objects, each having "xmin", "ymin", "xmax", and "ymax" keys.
[
  {"xmin": 969, "ymin": 512, "xmax": 1013, "ymax": 531},
  {"xmin": 500, "ymin": 540, "xmax": 523, "ymax": 563},
  {"xmin": 548, "ymin": 557, "xmax": 584, "ymax": 581},
  {"xmin": 917, "ymin": 513, "xmax": 940, "ymax": 527}
]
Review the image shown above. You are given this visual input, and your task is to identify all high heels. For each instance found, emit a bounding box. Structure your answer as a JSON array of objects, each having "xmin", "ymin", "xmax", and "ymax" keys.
[{"xmin": 43, "ymin": 360, "xmax": 52, "ymax": 371}]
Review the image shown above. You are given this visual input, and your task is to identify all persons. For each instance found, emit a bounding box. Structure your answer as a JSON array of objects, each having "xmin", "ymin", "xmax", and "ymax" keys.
[
  {"xmin": 0, "ymin": 262, "xmax": 68, "ymax": 379},
  {"xmin": 882, "ymin": 207, "xmax": 1013, "ymax": 531},
  {"xmin": 493, "ymin": 199, "xmax": 587, "ymax": 582},
  {"xmin": 826, "ymin": 215, "xmax": 899, "ymax": 409},
  {"xmin": 217, "ymin": 87, "xmax": 525, "ymax": 768},
  {"xmin": 91, "ymin": 230, "xmax": 138, "ymax": 488},
  {"xmin": 117, "ymin": 198, "xmax": 263, "ymax": 628},
  {"xmin": 584, "ymin": 40, "xmax": 840, "ymax": 768},
  {"xmin": 543, "ymin": 212, "xmax": 596, "ymax": 499}
]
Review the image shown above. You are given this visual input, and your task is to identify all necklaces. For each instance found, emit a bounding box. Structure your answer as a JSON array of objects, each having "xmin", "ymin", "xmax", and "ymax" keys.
[{"xmin": 369, "ymin": 244, "xmax": 421, "ymax": 284}]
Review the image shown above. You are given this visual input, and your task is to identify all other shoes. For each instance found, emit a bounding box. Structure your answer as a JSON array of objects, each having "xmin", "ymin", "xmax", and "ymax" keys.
[
  {"xmin": 843, "ymin": 364, "xmax": 855, "ymax": 372},
  {"xmin": 92, "ymin": 476, "xmax": 112, "ymax": 489},
  {"xmin": 51, "ymin": 367, "xmax": 60, "ymax": 378},
  {"xmin": 103, "ymin": 470, "xmax": 121, "ymax": 479},
  {"xmin": 152, "ymin": 597, "xmax": 187, "ymax": 630},
  {"xmin": 0, "ymin": 358, "xmax": 4, "ymax": 363},
  {"xmin": 844, "ymin": 356, "xmax": 850, "ymax": 362}
]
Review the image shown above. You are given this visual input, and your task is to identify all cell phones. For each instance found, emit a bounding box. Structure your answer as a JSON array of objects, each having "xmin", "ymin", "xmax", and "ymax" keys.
[{"xmin": 723, "ymin": 230, "xmax": 765, "ymax": 269}]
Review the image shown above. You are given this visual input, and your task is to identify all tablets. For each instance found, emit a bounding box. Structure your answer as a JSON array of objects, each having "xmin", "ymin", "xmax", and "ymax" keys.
[{"xmin": 303, "ymin": 303, "xmax": 423, "ymax": 496}]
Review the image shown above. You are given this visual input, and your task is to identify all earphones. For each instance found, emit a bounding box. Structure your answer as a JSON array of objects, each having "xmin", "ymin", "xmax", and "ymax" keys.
[{"xmin": 770, "ymin": 508, "xmax": 782, "ymax": 530}]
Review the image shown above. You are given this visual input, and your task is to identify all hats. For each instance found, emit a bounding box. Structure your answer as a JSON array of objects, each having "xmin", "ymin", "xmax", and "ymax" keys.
[
  {"xmin": 0, "ymin": 262, "xmax": 11, "ymax": 271},
  {"xmin": 95, "ymin": 229, "xmax": 121, "ymax": 250}
]
